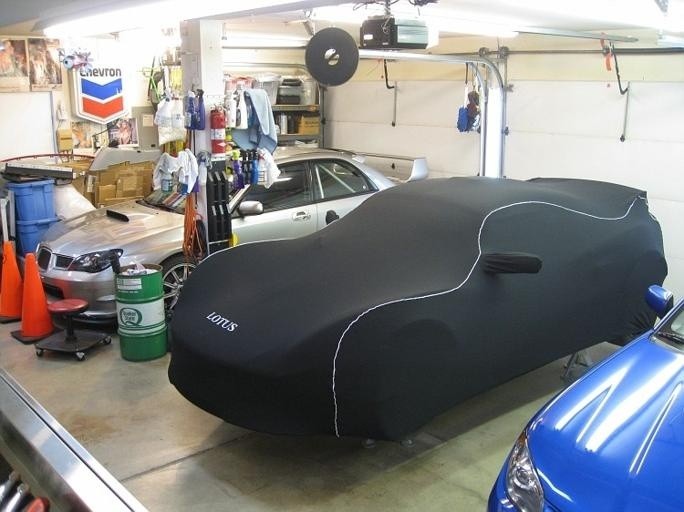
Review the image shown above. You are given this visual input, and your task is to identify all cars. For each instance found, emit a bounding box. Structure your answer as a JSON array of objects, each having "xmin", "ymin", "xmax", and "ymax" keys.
[
  {"xmin": 487, "ymin": 285, "xmax": 684, "ymax": 510},
  {"xmin": 34, "ymin": 147, "xmax": 430, "ymax": 331}
]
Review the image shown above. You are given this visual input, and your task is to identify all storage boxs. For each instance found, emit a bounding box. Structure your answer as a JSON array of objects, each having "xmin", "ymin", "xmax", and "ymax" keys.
[{"xmin": 272, "ymin": 110, "xmax": 321, "ymax": 136}]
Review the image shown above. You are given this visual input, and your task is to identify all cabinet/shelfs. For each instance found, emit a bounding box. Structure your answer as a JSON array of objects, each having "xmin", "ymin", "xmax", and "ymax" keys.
[{"xmin": 265, "ymin": 102, "xmax": 322, "ymax": 148}]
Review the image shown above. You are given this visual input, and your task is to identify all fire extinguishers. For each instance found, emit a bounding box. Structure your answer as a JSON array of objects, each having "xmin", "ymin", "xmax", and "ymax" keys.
[{"xmin": 211, "ymin": 103, "xmax": 227, "ymax": 154}]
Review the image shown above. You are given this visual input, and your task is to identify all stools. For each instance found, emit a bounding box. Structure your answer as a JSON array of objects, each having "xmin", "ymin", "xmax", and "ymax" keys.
[{"xmin": 30, "ymin": 298, "xmax": 113, "ymax": 362}]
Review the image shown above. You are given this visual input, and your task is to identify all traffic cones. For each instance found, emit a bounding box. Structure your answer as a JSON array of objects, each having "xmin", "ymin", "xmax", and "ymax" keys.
[
  {"xmin": 0, "ymin": 240, "xmax": 24, "ymax": 324},
  {"xmin": 11, "ymin": 252, "xmax": 63, "ymax": 346}
]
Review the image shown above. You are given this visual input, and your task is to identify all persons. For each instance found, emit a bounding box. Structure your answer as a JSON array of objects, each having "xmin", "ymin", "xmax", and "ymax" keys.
[{"xmin": 116, "ymin": 120, "xmax": 132, "ymax": 143}]
